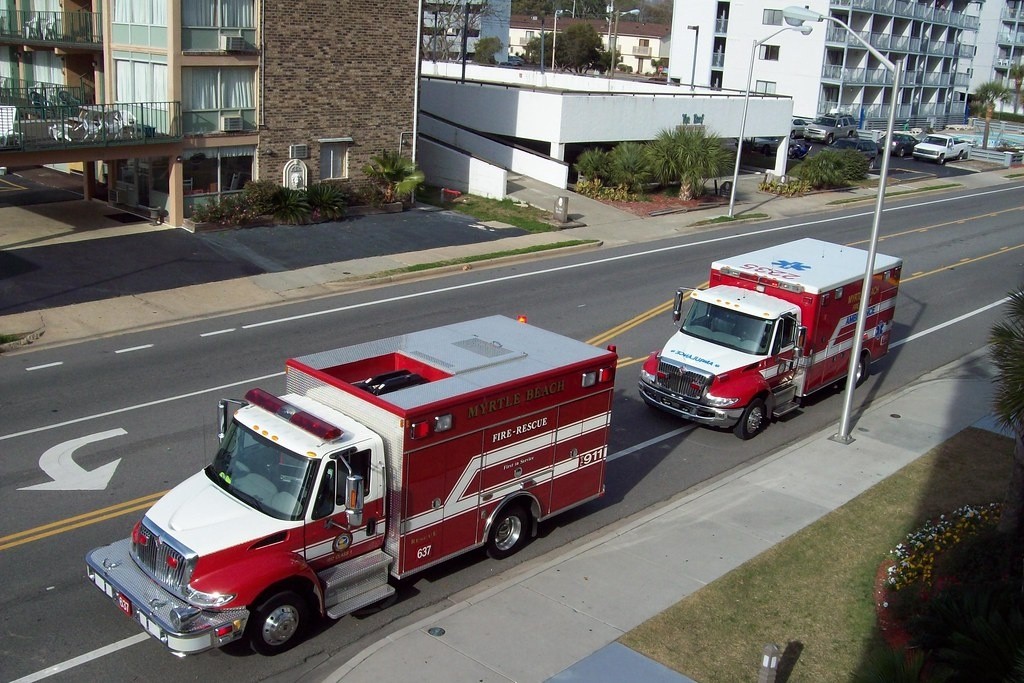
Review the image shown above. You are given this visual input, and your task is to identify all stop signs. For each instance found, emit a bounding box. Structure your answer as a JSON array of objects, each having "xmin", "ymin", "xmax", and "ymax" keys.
[{"xmin": 658, "ymin": 66, "xmax": 663, "ymax": 72}]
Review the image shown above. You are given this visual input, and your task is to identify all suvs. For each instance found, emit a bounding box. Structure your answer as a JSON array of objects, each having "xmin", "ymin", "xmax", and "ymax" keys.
[
  {"xmin": 803, "ymin": 113, "xmax": 858, "ymax": 145},
  {"xmin": 821, "ymin": 138, "xmax": 879, "ymax": 169}
]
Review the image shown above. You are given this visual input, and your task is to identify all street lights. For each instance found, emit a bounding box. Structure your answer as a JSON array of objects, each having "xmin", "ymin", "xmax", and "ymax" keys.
[
  {"xmin": 781, "ymin": 5, "xmax": 900, "ymax": 443},
  {"xmin": 611, "ymin": 10, "xmax": 640, "ymax": 78},
  {"xmin": 565, "ymin": 10, "xmax": 574, "ymax": 18},
  {"xmin": 727, "ymin": 25, "xmax": 815, "ymax": 217},
  {"xmin": 432, "ymin": 9, "xmax": 441, "ymax": 62},
  {"xmin": 531, "ymin": 15, "xmax": 545, "ymax": 74},
  {"xmin": 687, "ymin": 25, "xmax": 701, "ymax": 91}
]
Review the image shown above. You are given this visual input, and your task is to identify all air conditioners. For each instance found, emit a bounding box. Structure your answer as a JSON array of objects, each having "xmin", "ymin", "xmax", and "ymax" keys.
[
  {"xmin": 220, "ymin": 35, "xmax": 244, "ymax": 50},
  {"xmin": 220, "ymin": 115, "xmax": 243, "ymax": 132},
  {"xmin": 108, "ymin": 188, "xmax": 125, "ymax": 204}
]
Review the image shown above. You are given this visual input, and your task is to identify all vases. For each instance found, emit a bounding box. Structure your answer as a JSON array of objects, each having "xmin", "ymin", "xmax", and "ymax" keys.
[{"xmin": 181, "ymin": 217, "xmax": 270, "ymax": 231}]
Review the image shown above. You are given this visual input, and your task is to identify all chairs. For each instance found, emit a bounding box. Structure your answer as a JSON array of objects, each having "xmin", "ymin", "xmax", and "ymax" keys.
[
  {"xmin": 183, "ymin": 177, "xmax": 193, "ymax": 191},
  {"xmin": 711, "ymin": 308, "xmax": 736, "ymax": 335},
  {"xmin": 24, "ymin": 11, "xmax": 56, "ymax": 40},
  {"xmin": 222, "ymin": 172, "xmax": 240, "ymax": 192},
  {"xmin": 0, "ymin": 106, "xmax": 23, "ymax": 147},
  {"xmin": 58, "ymin": 91, "xmax": 81, "ymax": 117},
  {"xmin": 30, "ymin": 91, "xmax": 56, "ymax": 120},
  {"xmin": 257, "ymin": 446, "xmax": 281, "ymax": 484}
]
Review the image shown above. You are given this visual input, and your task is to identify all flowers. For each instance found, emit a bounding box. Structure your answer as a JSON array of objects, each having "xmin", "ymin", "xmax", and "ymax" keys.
[{"xmin": 190, "ymin": 193, "xmax": 256, "ymax": 230}]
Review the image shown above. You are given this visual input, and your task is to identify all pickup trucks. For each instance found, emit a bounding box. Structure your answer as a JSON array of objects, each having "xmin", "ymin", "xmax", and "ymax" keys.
[{"xmin": 912, "ymin": 134, "xmax": 969, "ymax": 165}]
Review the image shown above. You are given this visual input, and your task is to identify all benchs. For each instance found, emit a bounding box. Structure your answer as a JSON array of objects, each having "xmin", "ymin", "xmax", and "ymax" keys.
[{"xmin": 68, "ymin": 116, "xmax": 98, "ymax": 125}]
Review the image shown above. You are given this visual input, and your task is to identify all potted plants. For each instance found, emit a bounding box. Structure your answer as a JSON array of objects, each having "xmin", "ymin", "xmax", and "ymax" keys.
[
  {"xmin": 348, "ymin": 148, "xmax": 419, "ymax": 216},
  {"xmin": 269, "ymin": 184, "xmax": 347, "ymax": 225},
  {"xmin": 735, "ymin": 138, "xmax": 758, "ymax": 152}
]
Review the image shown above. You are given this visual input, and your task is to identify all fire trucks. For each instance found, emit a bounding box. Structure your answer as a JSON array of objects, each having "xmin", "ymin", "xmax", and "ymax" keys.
[
  {"xmin": 636, "ymin": 237, "xmax": 904, "ymax": 443},
  {"xmin": 84, "ymin": 314, "xmax": 620, "ymax": 659}
]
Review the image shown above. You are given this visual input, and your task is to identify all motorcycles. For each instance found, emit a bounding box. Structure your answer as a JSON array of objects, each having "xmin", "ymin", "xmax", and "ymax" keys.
[{"xmin": 790, "ymin": 144, "xmax": 813, "ymax": 161}]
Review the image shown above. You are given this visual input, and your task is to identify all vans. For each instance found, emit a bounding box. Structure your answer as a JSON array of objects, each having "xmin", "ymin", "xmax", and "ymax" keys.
[{"xmin": 501, "ymin": 56, "xmax": 524, "ymax": 66}]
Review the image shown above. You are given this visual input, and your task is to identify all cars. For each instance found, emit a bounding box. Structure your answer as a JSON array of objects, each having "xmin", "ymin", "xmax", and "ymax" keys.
[
  {"xmin": 875, "ymin": 133, "xmax": 920, "ymax": 157},
  {"xmin": 735, "ymin": 138, "xmax": 795, "ymax": 156},
  {"xmin": 791, "ymin": 118, "xmax": 809, "ymax": 139}
]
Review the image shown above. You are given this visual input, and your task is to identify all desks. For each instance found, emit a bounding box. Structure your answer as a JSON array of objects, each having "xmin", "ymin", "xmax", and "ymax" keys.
[{"xmin": 78, "ymin": 105, "xmax": 118, "ymax": 115}]
